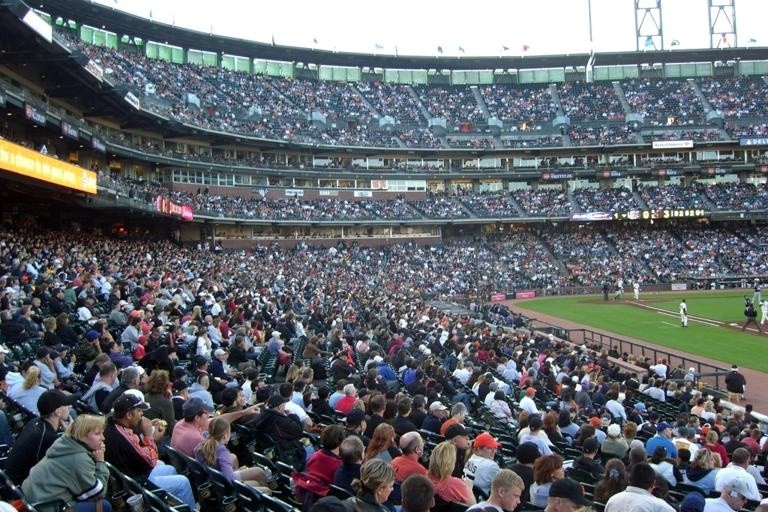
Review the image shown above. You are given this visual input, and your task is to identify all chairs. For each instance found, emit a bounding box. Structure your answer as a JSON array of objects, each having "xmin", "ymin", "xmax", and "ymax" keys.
[{"xmin": 0, "ymin": 304, "xmax": 696, "ymax": 512}]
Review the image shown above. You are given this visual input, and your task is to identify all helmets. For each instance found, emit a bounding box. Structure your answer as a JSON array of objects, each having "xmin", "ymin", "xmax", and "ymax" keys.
[{"xmin": 715, "ymin": 466, "xmax": 759, "ymax": 501}]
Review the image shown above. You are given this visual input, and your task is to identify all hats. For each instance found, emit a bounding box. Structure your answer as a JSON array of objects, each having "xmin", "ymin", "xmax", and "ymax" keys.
[
  {"xmin": 119, "ymin": 300, "xmax": 129, "ymax": 305},
  {"xmin": 530, "ymin": 417, "xmax": 601, "ymax": 453},
  {"xmin": 658, "ymin": 423, "xmax": 672, "ymax": 430},
  {"xmin": 682, "ymin": 491, "xmax": 706, "ymax": 511},
  {"xmin": 214, "ymin": 349, "xmax": 228, "ymax": 357},
  {"xmin": 549, "ymin": 478, "xmax": 593, "ymax": 506},
  {"xmin": 429, "ymin": 400, "xmax": 503, "ymax": 449},
  {"xmin": 37, "ymin": 389, "xmax": 152, "ymax": 415},
  {"xmin": 182, "ymin": 397, "xmax": 215, "ymax": 417}
]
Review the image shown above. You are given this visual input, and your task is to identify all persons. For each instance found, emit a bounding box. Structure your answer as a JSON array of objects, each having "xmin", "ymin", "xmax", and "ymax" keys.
[{"xmin": 0, "ymin": 32, "xmax": 766, "ymax": 511}]
